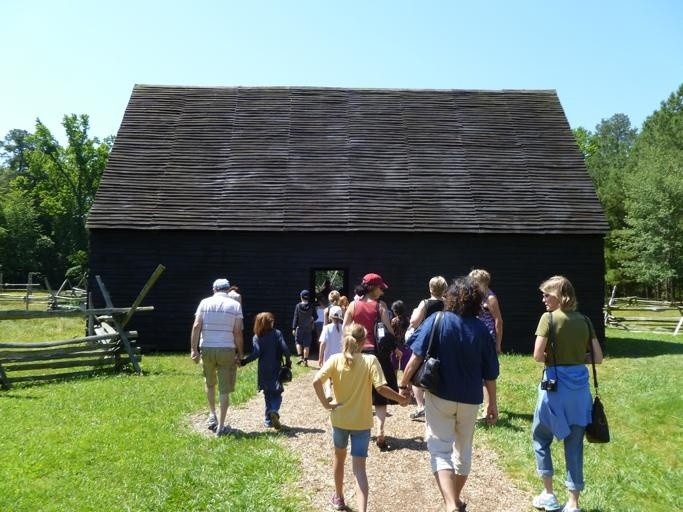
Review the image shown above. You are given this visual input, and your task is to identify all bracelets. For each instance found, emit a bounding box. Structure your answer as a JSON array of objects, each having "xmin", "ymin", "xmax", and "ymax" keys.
[{"xmin": 398, "ymin": 386, "xmax": 407, "ymax": 389}]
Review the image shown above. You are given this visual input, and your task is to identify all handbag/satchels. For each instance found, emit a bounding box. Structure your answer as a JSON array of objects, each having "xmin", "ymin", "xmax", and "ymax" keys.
[
  {"xmin": 586, "ymin": 394, "xmax": 610, "ymax": 443},
  {"xmin": 405, "ymin": 325, "xmax": 415, "ymax": 343},
  {"xmin": 411, "ymin": 358, "xmax": 441, "ymax": 390},
  {"xmin": 374, "ymin": 322, "xmax": 396, "ymax": 353},
  {"xmin": 277, "ymin": 365, "xmax": 292, "ymax": 384}
]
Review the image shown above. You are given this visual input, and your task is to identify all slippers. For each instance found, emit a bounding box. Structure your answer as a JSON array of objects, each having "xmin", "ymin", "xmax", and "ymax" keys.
[{"xmin": 376, "ymin": 440, "xmax": 387, "ymax": 451}]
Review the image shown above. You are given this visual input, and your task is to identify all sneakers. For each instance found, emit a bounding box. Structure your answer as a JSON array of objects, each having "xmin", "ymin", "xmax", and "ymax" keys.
[
  {"xmin": 207, "ymin": 415, "xmax": 218, "ymax": 429},
  {"xmin": 296, "ymin": 359, "xmax": 303, "ymax": 364},
  {"xmin": 217, "ymin": 426, "xmax": 230, "ymax": 437},
  {"xmin": 561, "ymin": 501, "xmax": 581, "ymax": 512},
  {"xmin": 264, "ymin": 420, "xmax": 271, "ymax": 426},
  {"xmin": 486, "ymin": 405, "xmax": 497, "ymax": 425},
  {"xmin": 533, "ymin": 492, "xmax": 559, "ymax": 511},
  {"xmin": 410, "ymin": 409, "xmax": 425, "ymax": 419},
  {"xmin": 330, "ymin": 493, "xmax": 345, "ymax": 510},
  {"xmin": 270, "ymin": 413, "xmax": 281, "ymax": 429},
  {"xmin": 303, "ymin": 361, "xmax": 308, "ymax": 367}
]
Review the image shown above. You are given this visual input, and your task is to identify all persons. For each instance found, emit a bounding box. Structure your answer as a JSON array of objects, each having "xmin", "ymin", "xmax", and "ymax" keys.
[
  {"xmin": 315, "ymin": 280, "xmax": 349, "ymax": 325},
  {"xmin": 190, "ymin": 278, "xmax": 243, "ymax": 436},
  {"xmin": 312, "ymin": 323, "xmax": 406, "ymax": 512},
  {"xmin": 399, "ymin": 278, "xmax": 499, "ymax": 512},
  {"xmin": 391, "ymin": 300, "xmax": 409, "ymax": 346},
  {"xmin": 318, "ymin": 305, "xmax": 343, "ymax": 368},
  {"xmin": 409, "ymin": 276, "xmax": 448, "ymax": 419},
  {"xmin": 291, "ymin": 290, "xmax": 317, "ymax": 367},
  {"xmin": 341, "ymin": 273, "xmax": 398, "ymax": 447},
  {"xmin": 227, "ymin": 285, "xmax": 241, "ymax": 304},
  {"xmin": 235, "ymin": 312, "xmax": 290, "ymax": 428},
  {"xmin": 469, "ymin": 269, "xmax": 503, "ymax": 420},
  {"xmin": 531, "ymin": 275, "xmax": 603, "ymax": 512}
]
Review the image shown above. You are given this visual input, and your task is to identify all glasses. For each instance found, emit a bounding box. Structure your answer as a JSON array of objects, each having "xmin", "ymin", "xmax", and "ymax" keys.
[{"xmin": 542, "ymin": 293, "xmax": 550, "ymax": 298}]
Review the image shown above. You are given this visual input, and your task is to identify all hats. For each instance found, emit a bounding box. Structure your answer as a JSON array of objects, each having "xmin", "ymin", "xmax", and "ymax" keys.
[
  {"xmin": 213, "ymin": 278, "xmax": 230, "ymax": 290},
  {"xmin": 330, "ymin": 305, "xmax": 344, "ymax": 321},
  {"xmin": 300, "ymin": 290, "xmax": 310, "ymax": 297},
  {"xmin": 362, "ymin": 273, "xmax": 388, "ymax": 289}
]
